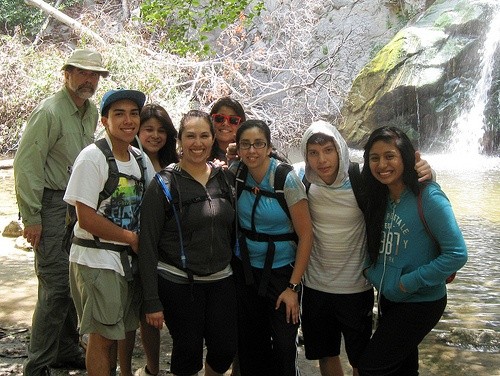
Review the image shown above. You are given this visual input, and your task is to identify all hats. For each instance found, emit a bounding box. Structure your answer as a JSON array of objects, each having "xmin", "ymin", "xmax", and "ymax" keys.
[
  {"xmin": 101, "ymin": 88, "xmax": 146, "ymax": 115},
  {"xmin": 61, "ymin": 51, "xmax": 109, "ymax": 78}
]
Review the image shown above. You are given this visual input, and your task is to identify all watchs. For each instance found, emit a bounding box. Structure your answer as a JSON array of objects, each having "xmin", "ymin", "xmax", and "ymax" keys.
[{"xmin": 287, "ymin": 281, "xmax": 301, "ymax": 292}]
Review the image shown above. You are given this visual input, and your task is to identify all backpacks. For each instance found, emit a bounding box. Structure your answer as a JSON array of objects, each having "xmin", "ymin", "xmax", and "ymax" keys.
[{"xmin": 61, "ymin": 139, "xmax": 146, "ymax": 254}]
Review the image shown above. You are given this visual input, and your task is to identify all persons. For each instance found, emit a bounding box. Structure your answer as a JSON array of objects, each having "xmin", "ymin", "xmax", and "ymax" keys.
[
  {"xmin": 13, "ymin": 49, "xmax": 111, "ymax": 376},
  {"xmin": 62, "ymin": 88, "xmax": 468, "ymax": 376}
]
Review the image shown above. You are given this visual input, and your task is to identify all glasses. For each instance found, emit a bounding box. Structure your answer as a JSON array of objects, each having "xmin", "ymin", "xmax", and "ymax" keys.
[
  {"xmin": 238, "ymin": 142, "xmax": 266, "ymax": 150},
  {"xmin": 212, "ymin": 114, "xmax": 241, "ymax": 126}
]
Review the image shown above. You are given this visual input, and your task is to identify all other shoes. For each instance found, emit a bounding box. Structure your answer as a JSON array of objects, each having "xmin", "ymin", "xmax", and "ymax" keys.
[
  {"xmin": 134, "ymin": 364, "xmax": 153, "ymax": 376},
  {"xmin": 49, "ymin": 347, "xmax": 85, "ymax": 369}
]
